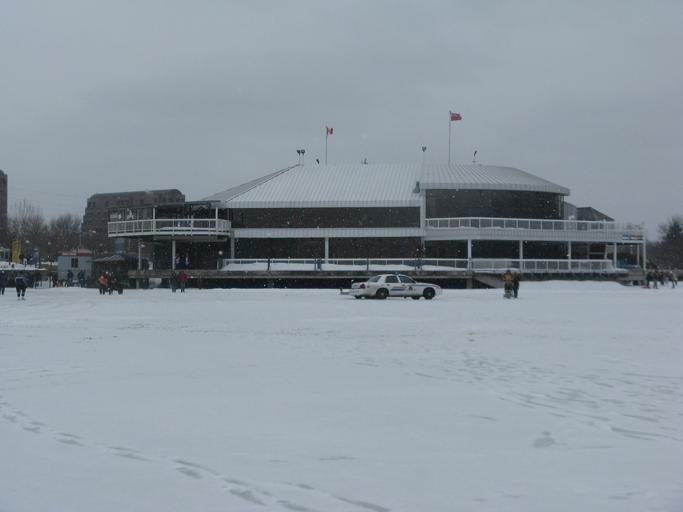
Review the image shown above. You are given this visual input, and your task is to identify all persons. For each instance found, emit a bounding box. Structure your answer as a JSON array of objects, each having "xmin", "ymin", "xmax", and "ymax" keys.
[
  {"xmin": 95, "ymin": 273, "xmax": 109, "ymax": 295},
  {"xmin": 168, "ymin": 271, "xmax": 178, "ymax": 293},
  {"xmin": 501, "ymin": 268, "xmax": 513, "ymax": 299},
  {"xmin": 106, "ymin": 271, "xmax": 117, "ymax": 296},
  {"xmin": 117, "ymin": 271, "xmax": 124, "ymax": 295},
  {"xmin": 176, "ymin": 268, "xmax": 188, "ymax": 293},
  {"xmin": 645, "ymin": 270, "xmax": 682, "ymax": 289},
  {"xmin": 0, "ymin": 269, "xmax": 5, "ymax": 296},
  {"xmin": 14, "ymin": 273, "xmax": 26, "ymax": 300},
  {"xmin": 18, "ymin": 253, "xmax": 149, "ymax": 287},
  {"xmin": 512, "ymin": 271, "xmax": 518, "ymax": 298}
]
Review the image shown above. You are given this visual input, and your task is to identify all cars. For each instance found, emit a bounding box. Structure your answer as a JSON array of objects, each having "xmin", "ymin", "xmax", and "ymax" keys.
[{"xmin": 350, "ymin": 272, "xmax": 442, "ymax": 300}]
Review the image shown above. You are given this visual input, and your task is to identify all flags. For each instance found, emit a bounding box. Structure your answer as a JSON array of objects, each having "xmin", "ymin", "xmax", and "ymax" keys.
[
  {"xmin": 450, "ymin": 114, "xmax": 462, "ymax": 121},
  {"xmin": 327, "ymin": 127, "xmax": 333, "ymax": 135}
]
